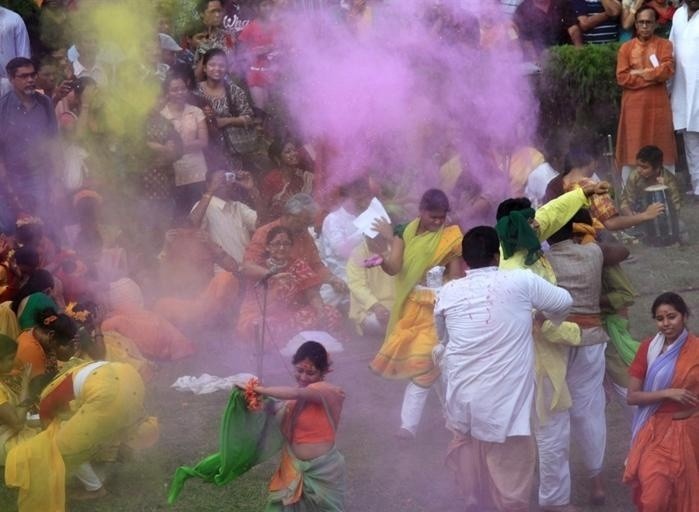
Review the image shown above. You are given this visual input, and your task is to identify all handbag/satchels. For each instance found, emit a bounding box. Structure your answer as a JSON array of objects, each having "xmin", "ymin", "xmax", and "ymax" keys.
[{"xmin": 221, "ymin": 124, "xmax": 258, "ymax": 156}]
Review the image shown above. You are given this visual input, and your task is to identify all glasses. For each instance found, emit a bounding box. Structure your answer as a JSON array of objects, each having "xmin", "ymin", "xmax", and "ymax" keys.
[{"xmin": 12, "ymin": 71, "xmax": 39, "ymax": 82}]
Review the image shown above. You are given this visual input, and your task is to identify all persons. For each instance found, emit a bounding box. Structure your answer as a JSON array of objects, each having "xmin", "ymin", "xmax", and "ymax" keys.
[{"xmin": 1, "ymin": 1, "xmax": 698, "ymax": 512}]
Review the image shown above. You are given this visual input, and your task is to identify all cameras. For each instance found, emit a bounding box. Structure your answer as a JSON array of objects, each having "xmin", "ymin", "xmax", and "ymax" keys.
[
  {"xmin": 222, "ymin": 172, "xmax": 237, "ymax": 183},
  {"xmin": 66, "ymin": 80, "xmax": 84, "ymax": 91}
]
